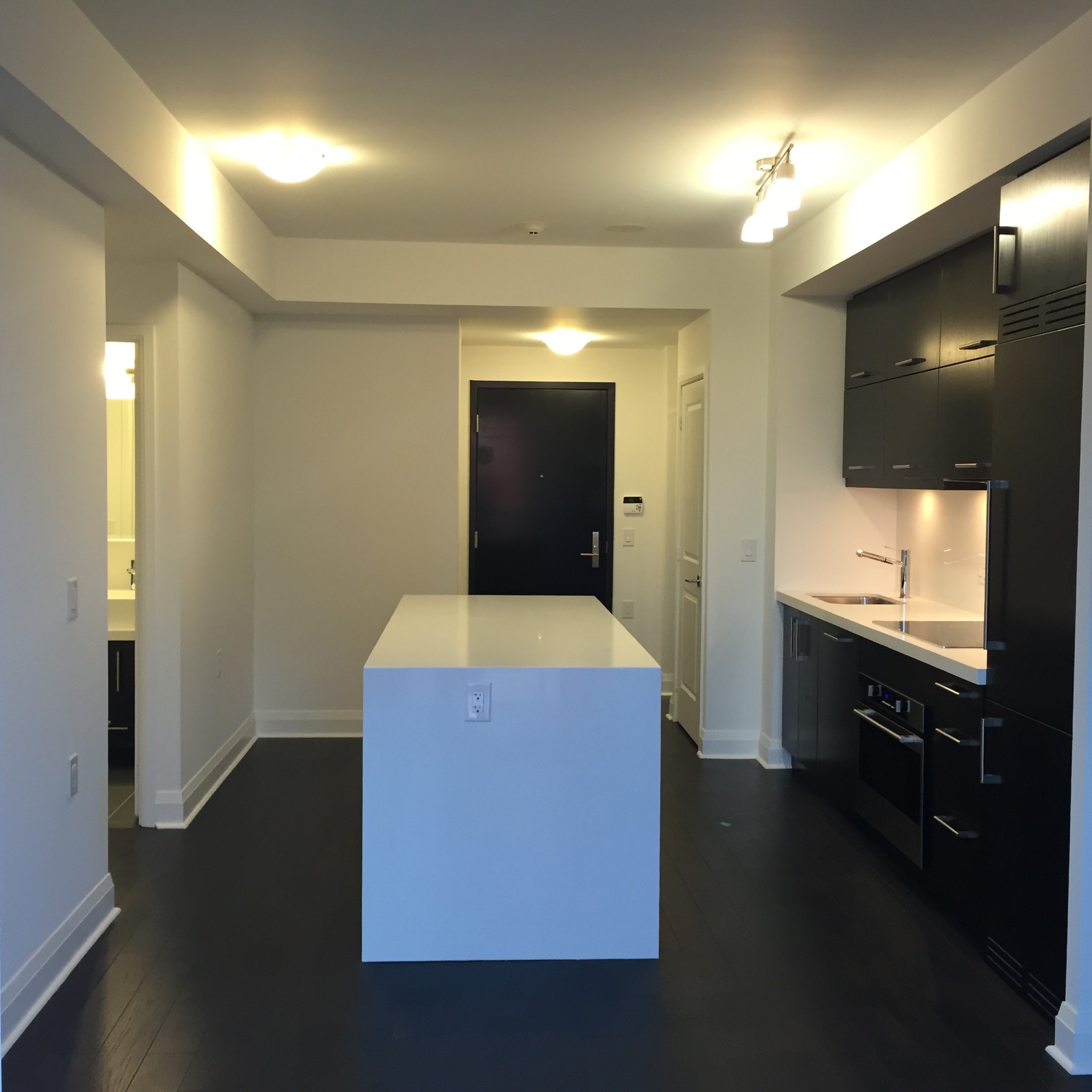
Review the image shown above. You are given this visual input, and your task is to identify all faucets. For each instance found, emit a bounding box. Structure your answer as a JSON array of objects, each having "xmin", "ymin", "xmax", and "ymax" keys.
[{"xmin": 856, "ymin": 548, "xmax": 912, "ymax": 599}]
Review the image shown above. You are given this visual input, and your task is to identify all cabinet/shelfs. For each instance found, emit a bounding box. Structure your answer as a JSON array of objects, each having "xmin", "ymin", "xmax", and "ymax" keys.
[{"xmin": 783, "ymin": 137, "xmax": 1090, "ymax": 1018}]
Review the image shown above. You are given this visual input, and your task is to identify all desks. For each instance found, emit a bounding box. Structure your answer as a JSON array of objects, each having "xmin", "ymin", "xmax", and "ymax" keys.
[{"xmin": 361, "ymin": 594, "xmax": 661, "ymax": 962}]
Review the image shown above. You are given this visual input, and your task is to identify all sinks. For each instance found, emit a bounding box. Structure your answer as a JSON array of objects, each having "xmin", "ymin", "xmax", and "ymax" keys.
[{"xmin": 806, "ymin": 591, "xmax": 907, "ymax": 606}]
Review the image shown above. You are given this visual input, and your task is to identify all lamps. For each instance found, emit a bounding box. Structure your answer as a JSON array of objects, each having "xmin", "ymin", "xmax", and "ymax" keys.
[{"xmin": 741, "ymin": 144, "xmax": 801, "ymax": 243}]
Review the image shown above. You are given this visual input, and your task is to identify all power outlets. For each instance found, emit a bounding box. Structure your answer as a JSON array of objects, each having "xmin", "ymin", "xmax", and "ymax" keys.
[
  {"xmin": 623, "ymin": 600, "xmax": 633, "ymax": 618},
  {"xmin": 467, "ymin": 683, "xmax": 490, "ymax": 722}
]
